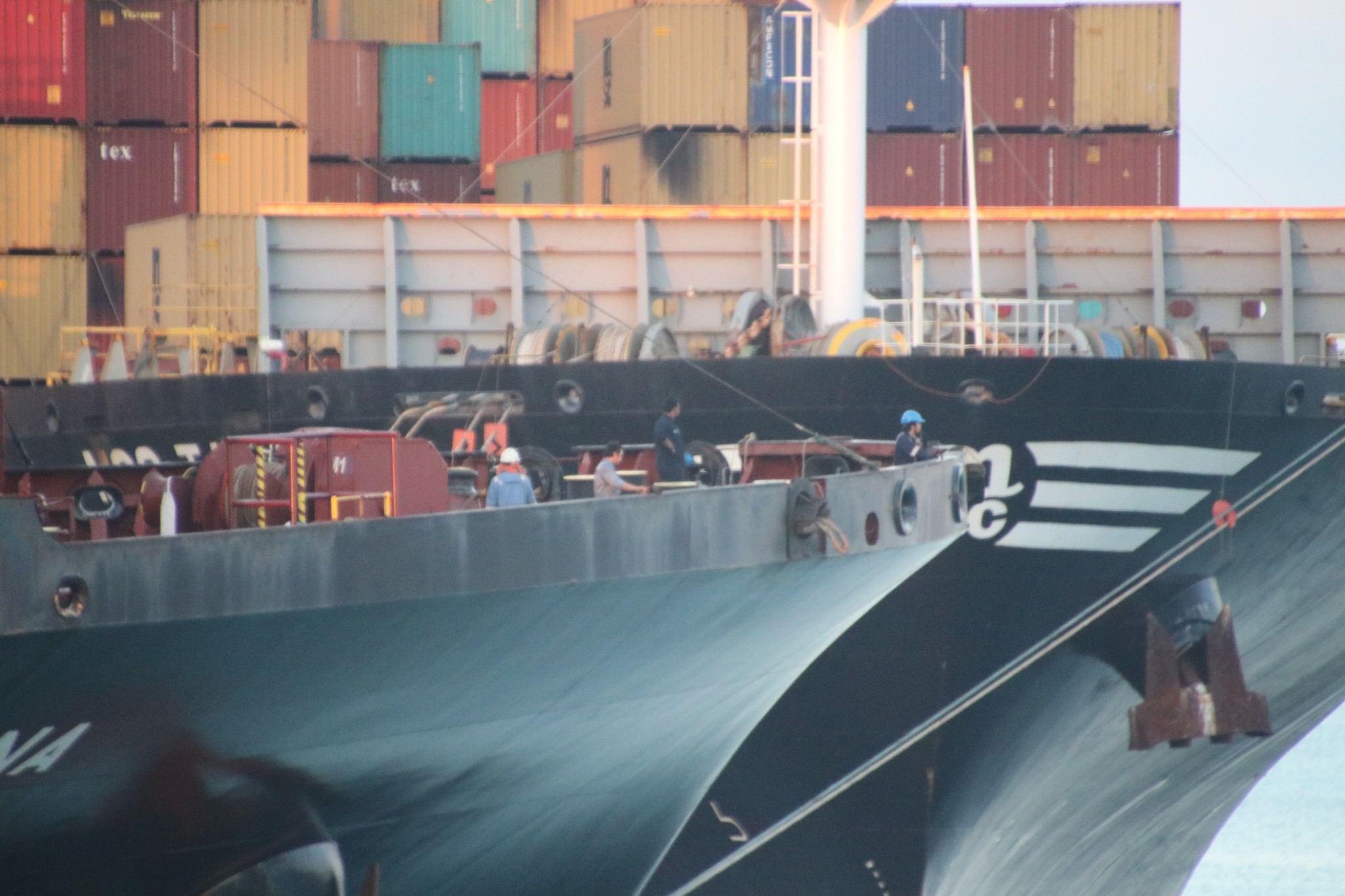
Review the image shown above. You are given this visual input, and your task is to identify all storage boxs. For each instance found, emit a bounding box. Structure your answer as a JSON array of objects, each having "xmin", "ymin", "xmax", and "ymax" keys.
[{"xmin": 0, "ymin": 0, "xmax": 1183, "ymax": 380}]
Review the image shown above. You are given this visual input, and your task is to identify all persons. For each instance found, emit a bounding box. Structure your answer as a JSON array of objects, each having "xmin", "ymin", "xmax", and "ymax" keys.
[
  {"xmin": 484, "ymin": 448, "xmax": 538, "ymax": 508},
  {"xmin": 653, "ymin": 398, "xmax": 693, "ymax": 482},
  {"xmin": 593, "ymin": 440, "xmax": 649, "ymax": 498},
  {"xmin": 893, "ymin": 409, "xmax": 940, "ymax": 465}
]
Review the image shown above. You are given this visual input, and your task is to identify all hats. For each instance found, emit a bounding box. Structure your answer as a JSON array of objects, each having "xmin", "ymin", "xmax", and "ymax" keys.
[
  {"xmin": 901, "ymin": 410, "xmax": 926, "ymax": 424},
  {"xmin": 499, "ymin": 447, "xmax": 520, "ymax": 464}
]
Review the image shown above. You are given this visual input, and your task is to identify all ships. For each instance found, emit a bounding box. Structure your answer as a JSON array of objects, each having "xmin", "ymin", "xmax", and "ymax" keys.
[{"xmin": 0, "ymin": 1, "xmax": 1345, "ymax": 895}]
